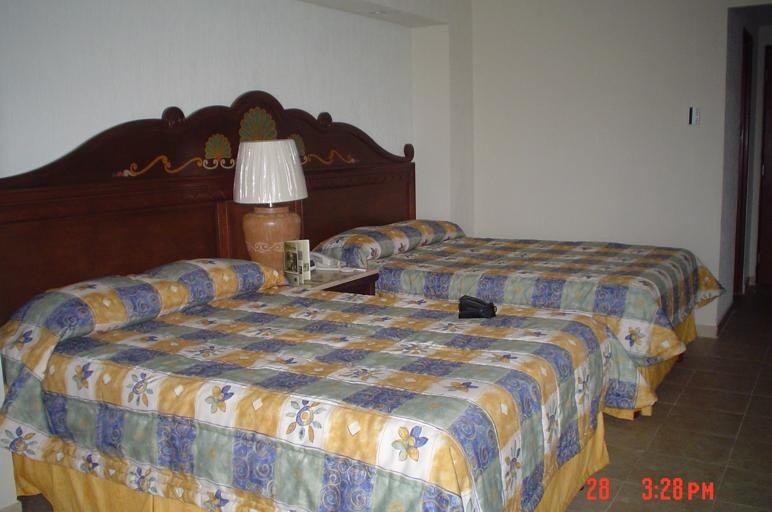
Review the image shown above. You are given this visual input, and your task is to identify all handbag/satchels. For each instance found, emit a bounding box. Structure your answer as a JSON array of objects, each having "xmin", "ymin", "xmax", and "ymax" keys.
[{"xmin": 458, "ymin": 295, "xmax": 497, "ymax": 318}]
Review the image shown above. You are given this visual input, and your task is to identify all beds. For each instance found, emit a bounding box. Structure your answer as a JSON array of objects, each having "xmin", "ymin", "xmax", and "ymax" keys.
[
  {"xmin": 311, "ymin": 220, "xmax": 726, "ymax": 421},
  {"xmin": 0, "ymin": 258, "xmax": 659, "ymax": 512}
]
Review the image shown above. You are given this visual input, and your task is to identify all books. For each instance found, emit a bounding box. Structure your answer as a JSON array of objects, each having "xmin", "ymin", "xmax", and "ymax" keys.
[{"xmin": 284, "ymin": 240, "xmax": 311, "ymax": 288}]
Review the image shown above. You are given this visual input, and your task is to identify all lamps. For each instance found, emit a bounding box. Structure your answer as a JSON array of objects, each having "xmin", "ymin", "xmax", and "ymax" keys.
[{"xmin": 233, "ymin": 140, "xmax": 309, "ymax": 275}]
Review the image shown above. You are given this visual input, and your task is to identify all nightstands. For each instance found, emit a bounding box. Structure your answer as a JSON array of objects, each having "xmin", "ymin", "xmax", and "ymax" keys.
[{"xmin": 296, "ymin": 269, "xmax": 379, "ymax": 295}]
